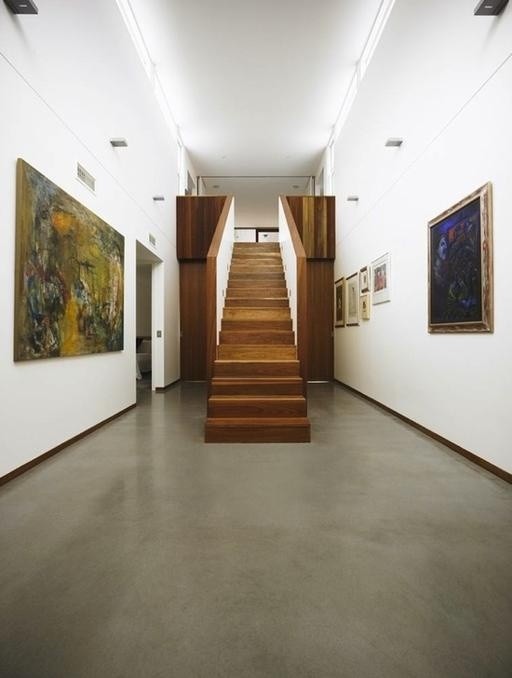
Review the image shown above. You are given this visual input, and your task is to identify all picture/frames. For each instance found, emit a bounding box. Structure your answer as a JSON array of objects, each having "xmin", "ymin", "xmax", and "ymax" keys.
[
  {"xmin": 333, "ymin": 248, "xmax": 391, "ymax": 329},
  {"xmin": 425, "ymin": 180, "xmax": 495, "ymax": 334}
]
